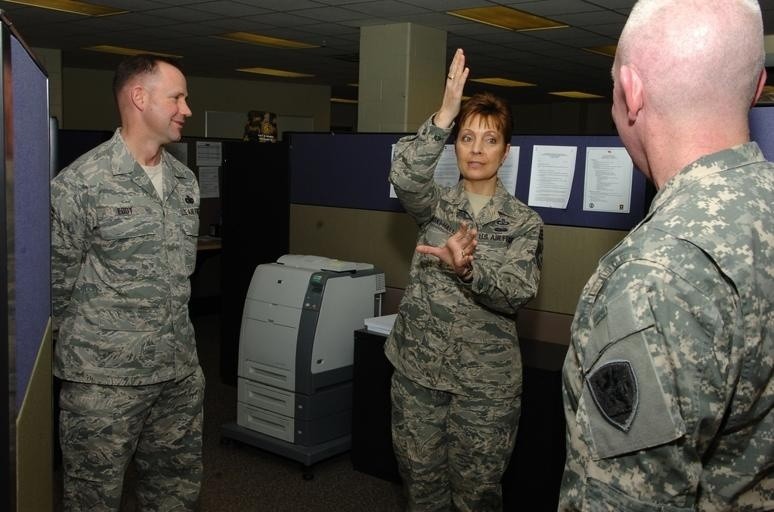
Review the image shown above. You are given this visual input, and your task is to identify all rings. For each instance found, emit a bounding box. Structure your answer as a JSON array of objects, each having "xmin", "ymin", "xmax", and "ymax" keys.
[{"xmin": 462, "ymin": 251, "xmax": 465, "ymax": 258}]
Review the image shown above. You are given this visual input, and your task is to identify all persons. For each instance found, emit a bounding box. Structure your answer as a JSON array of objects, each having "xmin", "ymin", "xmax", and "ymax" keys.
[
  {"xmin": 556, "ymin": 1, "xmax": 773, "ymax": 512},
  {"xmin": 387, "ymin": 48, "xmax": 544, "ymax": 511},
  {"xmin": 43, "ymin": 53, "xmax": 207, "ymax": 511}
]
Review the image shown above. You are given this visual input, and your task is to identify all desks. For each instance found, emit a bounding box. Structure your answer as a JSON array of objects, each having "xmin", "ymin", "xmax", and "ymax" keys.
[{"xmin": 350, "ymin": 328, "xmax": 568, "ymax": 511}]
